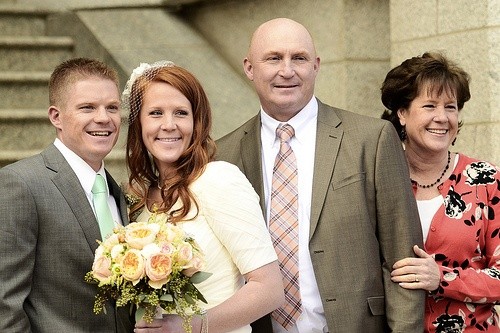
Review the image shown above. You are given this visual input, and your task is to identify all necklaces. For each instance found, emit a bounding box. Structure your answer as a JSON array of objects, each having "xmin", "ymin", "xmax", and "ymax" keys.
[
  {"xmin": 156, "ymin": 176, "xmax": 176, "ymax": 191},
  {"xmin": 403, "ymin": 149, "xmax": 451, "ymax": 189}
]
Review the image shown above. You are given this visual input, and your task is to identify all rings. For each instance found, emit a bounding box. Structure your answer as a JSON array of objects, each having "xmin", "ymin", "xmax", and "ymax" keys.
[{"xmin": 415, "ymin": 273, "xmax": 419, "ymax": 281}]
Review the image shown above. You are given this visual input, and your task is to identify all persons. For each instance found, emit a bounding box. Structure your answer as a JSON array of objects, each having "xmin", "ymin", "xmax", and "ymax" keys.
[
  {"xmin": 378, "ymin": 51, "xmax": 500, "ymax": 333},
  {"xmin": 117, "ymin": 60, "xmax": 286, "ymax": 333},
  {"xmin": 0, "ymin": 55, "xmax": 137, "ymax": 333},
  {"xmin": 207, "ymin": 16, "xmax": 428, "ymax": 333}
]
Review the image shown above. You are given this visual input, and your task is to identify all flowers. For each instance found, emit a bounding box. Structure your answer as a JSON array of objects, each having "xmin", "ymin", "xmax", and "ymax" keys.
[
  {"xmin": 122, "ymin": 60, "xmax": 152, "ymax": 97},
  {"xmin": 84, "ymin": 203, "xmax": 214, "ymax": 333},
  {"xmin": 119, "ymin": 180, "xmax": 142, "ymax": 206}
]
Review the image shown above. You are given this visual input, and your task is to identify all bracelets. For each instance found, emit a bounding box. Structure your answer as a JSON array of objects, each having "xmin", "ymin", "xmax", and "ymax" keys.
[{"xmin": 198, "ymin": 314, "xmax": 209, "ymax": 333}]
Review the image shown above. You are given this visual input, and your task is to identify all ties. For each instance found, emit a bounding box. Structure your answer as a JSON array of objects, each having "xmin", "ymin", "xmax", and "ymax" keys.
[
  {"xmin": 268, "ymin": 124, "xmax": 304, "ymax": 332},
  {"xmin": 90, "ymin": 174, "xmax": 116, "ymax": 244}
]
